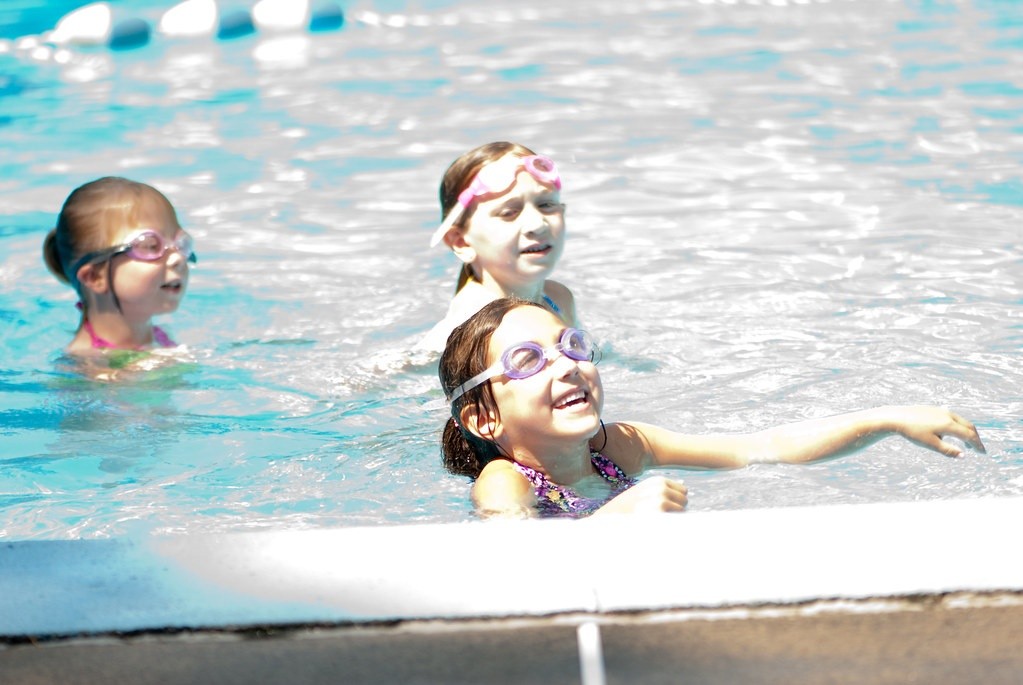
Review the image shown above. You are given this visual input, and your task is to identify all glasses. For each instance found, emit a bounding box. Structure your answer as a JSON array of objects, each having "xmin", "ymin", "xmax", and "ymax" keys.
[
  {"xmin": 428, "ymin": 154, "xmax": 561, "ymax": 248},
  {"xmin": 71, "ymin": 228, "xmax": 193, "ymax": 283},
  {"xmin": 448, "ymin": 326, "xmax": 594, "ymax": 403}
]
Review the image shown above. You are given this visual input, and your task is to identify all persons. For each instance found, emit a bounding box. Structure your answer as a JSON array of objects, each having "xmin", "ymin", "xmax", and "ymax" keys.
[
  {"xmin": 438, "ymin": 297, "xmax": 986, "ymax": 523},
  {"xmin": 420, "ymin": 140, "xmax": 577, "ymax": 361},
  {"xmin": 40, "ymin": 176, "xmax": 192, "ymax": 376}
]
